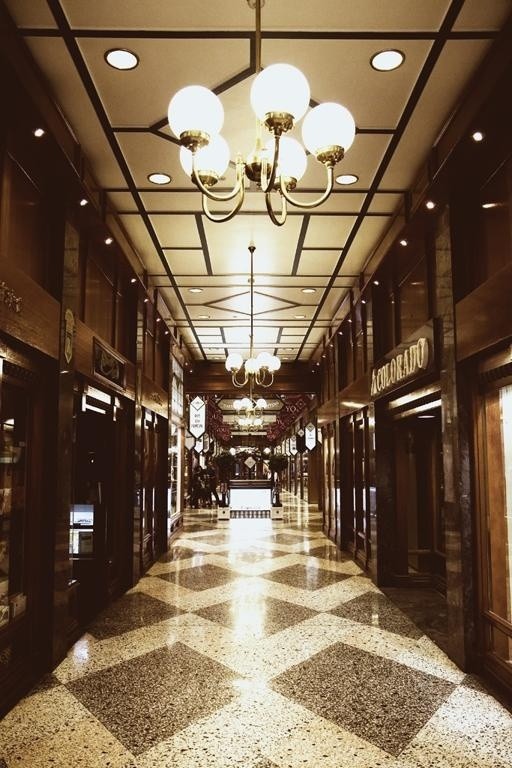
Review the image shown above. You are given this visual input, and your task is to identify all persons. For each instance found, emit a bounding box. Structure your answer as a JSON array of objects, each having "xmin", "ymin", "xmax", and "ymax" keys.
[{"xmin": 189, "ymin": 462, "xmax": 221, "ymax": 509}]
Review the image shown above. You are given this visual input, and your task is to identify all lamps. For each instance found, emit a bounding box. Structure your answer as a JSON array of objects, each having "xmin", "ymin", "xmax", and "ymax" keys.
[
  {"xmin": 224, "ymin": 245, "xmax": 281, "ymax": 388},
  {"xmin": 167, "ymin": 2, "xmax": 356, "ymax": 228}
]
